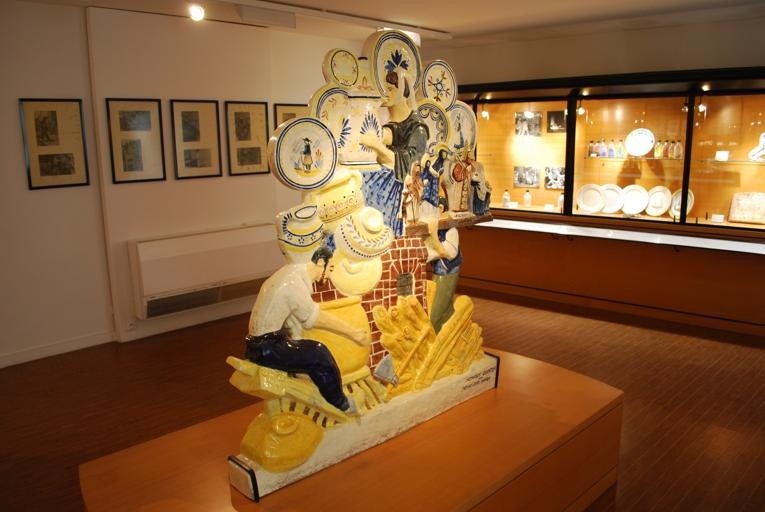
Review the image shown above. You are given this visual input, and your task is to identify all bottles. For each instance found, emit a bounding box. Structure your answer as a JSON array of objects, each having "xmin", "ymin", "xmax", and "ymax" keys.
[
  {"xmin": 524, "ymin": 190, "xmax": 532, "ymax": 208},
  {"xmin": 654, "ymin": 139, "xmax": 682, "ymax": 160},
  {"xmin": 589, "ymin": 140, "xmax": 623, "ymax": 158},
  {"xmin": 503, "ymin": 189, "xmax": 510, "ymax": 206},
  {"xmin": 558, "ymin": 193, "xmax": 564, "ymax": 214}
]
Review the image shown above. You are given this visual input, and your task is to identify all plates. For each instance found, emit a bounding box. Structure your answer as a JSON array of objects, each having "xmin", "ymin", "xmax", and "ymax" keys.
[
  {"xmin": 625, "ymin": 127, "xmax": 655, "ymax": 157},
  {"xmin": 577, "ymin": 183, "xmax": 694, "ymax": 219}
]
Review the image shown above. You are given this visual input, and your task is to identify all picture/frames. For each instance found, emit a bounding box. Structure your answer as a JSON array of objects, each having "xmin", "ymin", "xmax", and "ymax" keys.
[
  {"xmin": 547, "ymin": 111, "xmax": 567, "ymax": 132},
  {"xmin": 170, "ymin": 99, "xmax": 223, "ymax": 180},
  {"xmin": 225, "ymin": 101, "xmax": 270, "ymax": 176},
  {"xmin": 728, "ymin": 192, "xmax": 765, "ymax": 225},
  {"xmin": 18, "ymin": 98, "xmax": 90, "ymax": 190},
  {"xmin": 274, "ymin": 103, "xmax": 310, "ymax": 130},
  {"xmin": 105, "ymin": 98, "xmax": 166, "ymax": 184}
]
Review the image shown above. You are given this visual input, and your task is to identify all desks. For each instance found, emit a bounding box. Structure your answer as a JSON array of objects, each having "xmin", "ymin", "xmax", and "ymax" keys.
[{"xmin": 77, "ymin": 347, "xmax": 624, "ymax": 512}]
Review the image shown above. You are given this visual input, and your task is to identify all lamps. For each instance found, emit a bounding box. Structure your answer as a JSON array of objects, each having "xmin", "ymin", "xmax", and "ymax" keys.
[
  {"xmin": 698, "ymin": 95, "xmax": 706, "ymax": 113},
  {"xmin": 577, "ymin": 100, "xmax": 585, "ymax": 115},
  {"xmin": 524, "ymin": 103, "xmax": 534, "ymax": 118},
  {"xmin": 236, "ymin": 5, "xmax": 296, "ymax": 30},
  {"xmin": 376, "ymin": 26, "xmax": 421, "ymax": 49},
  {"xmin": 482, "ymin": 103, "xmax": 487, "ymax": 118},
  {"xmin": 680, "ymin": 97, "xmax": 688, "ymax": 113}
]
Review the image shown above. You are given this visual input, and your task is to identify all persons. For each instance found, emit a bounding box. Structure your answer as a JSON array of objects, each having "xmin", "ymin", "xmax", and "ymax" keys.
[
  {"xmin": 428, "ymin": 196, "xmax": 463, "ymax": 335},
  {"xmin": 300, "ymin": 137, "xmax": 313, "ymax": 173},
  {"xmin": 245, "ymin": 245, "xmax": 368, "ymax": 414},
  {"xmin": 398, "ymin": 148, "xmax": 491, "ymax": 225},
  {"xmin": 358, "ymin": 71, "xmax": 431, "ymax": 234}
]
